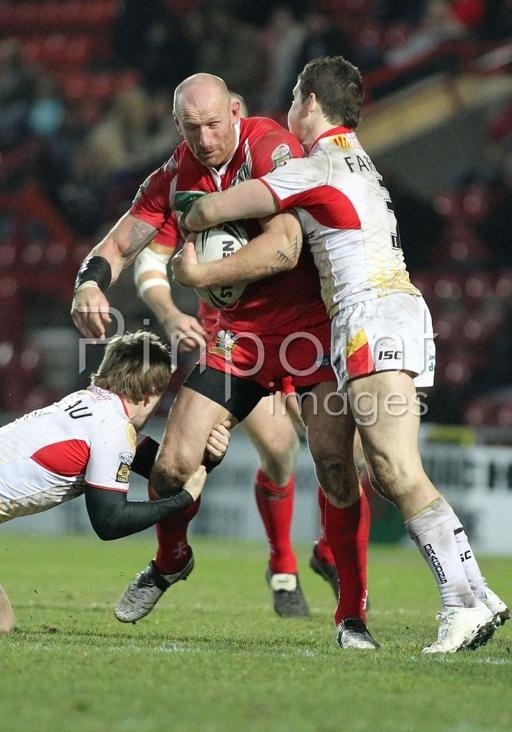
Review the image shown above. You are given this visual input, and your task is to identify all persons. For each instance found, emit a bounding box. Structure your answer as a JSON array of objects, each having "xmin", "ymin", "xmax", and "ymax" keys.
[
  {"xmin": 70, "ymin": 70, "xmax": 382, "ymax": 653},
  {"xmin": 3, "ymin": 330, "xmax": 231, "ymax": 633},
  {"xmin": 228, "ymin": 90, "xmax": 365, "ymax": 618},
  {"xmin": 181, "ymin": 56, "xmax": 511, "ymax": 656}
]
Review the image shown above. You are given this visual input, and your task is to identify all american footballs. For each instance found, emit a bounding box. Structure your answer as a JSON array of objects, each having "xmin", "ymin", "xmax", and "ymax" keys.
[{"xmin": 191, "ymin": 220, "xmax": 250, "ymax": 309}]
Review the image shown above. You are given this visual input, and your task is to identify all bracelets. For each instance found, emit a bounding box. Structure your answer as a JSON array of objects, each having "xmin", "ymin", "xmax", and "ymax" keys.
[
  {"xmin": 139, "ymin": 278, "xmax": 171, "ymax": 299},
  {"xmin": 74, "ymin": 255, "xmax": 111, "ymax": 293},
  {"xmin": 180, "ymin": 208, "xmax": 190, "ymax": 237}
]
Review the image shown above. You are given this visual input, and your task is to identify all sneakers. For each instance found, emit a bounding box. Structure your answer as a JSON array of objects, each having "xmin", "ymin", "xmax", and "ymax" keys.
[
  {"xmin": 114, "ymin": 544, "xmax": 194, "ymax": 624},
  {"xmin": 265, "ymin": 567, "xmax": 310, "ymax": 617},
  {"xmin": 310, "ymin": 541, "xmax": 380, "ymax": 651},
  {"xmin": 421, "ymin": 585, "xmax": 511, "ymax": 654}
]
